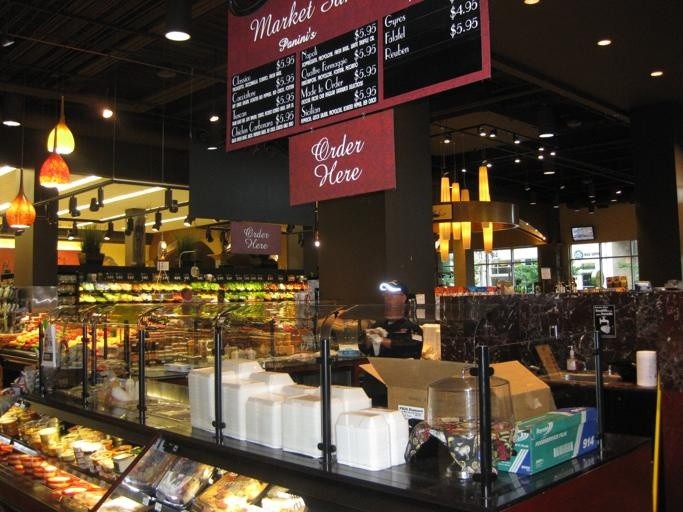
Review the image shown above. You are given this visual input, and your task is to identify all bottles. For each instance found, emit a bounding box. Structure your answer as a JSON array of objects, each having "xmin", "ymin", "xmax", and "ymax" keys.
[{"xmin": 111, "ymin": 374, "xmax": 139, "ymax": 402}]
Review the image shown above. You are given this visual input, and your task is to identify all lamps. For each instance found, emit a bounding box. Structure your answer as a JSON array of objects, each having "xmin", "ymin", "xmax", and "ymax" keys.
[
  {"xmin": 0, "ymin": 87, "xmax": 231, "ymax": 240},
  {"xmin": 429, "ymin": 123, "xmax": 555, "ymax": 262}
]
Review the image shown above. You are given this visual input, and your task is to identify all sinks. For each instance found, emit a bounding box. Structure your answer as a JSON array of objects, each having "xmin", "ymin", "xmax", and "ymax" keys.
[{"xmin": 541, "ymin": 372, "xmax": 620, "ymax": 384}]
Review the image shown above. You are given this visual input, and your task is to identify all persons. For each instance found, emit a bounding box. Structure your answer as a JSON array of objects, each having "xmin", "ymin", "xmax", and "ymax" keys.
[
  {"xmin": 179, "ymin": 287, "xmax": 195, "ymax": 303},
  {"xmin": 355, "ymin": 277, "xmax": 425, "ymax": 409},
  {"xmin": 209, "ymin": 289, "xmax": 229, "ymax": 302}
]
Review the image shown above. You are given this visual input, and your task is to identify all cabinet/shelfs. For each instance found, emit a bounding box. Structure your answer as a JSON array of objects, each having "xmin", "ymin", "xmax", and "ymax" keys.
[{"xmin": 57, "ymin": 271, "xmax": 307, "ymax": 310}]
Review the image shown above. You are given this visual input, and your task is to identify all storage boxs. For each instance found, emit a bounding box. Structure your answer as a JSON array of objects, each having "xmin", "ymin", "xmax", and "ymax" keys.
[{"xmin": 355, "ymin": 356, "xmax": 554, "ymax": 427}]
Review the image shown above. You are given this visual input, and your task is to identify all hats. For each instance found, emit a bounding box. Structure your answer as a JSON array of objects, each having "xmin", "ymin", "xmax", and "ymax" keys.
[{"xmin": 378, "ymin": 279, "xmax": 409, "ymax": 296}]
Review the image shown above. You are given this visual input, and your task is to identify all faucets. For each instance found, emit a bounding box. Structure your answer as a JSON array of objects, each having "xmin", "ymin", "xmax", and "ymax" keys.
[
  {"xmin": 566, "ymin": 345, "xmax": 579, "ymax": 370},
  {"xmin": 578, "ymin": 333, "xmax": 593, "ymax": 349}
]
